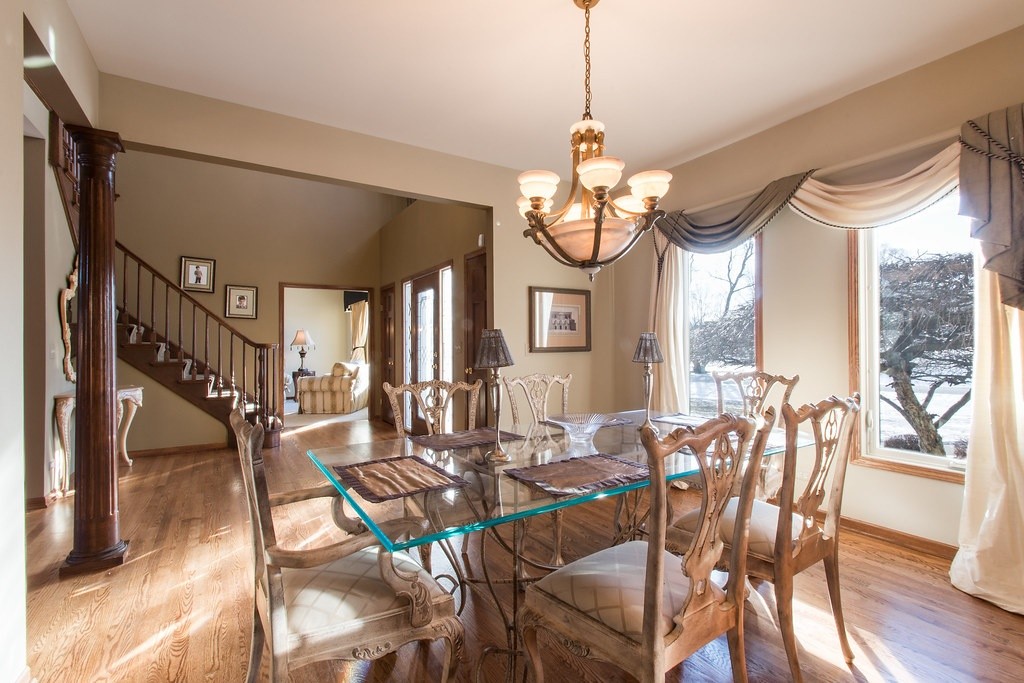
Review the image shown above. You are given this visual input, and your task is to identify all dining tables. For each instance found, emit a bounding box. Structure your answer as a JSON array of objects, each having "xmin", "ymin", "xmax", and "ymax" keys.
[{"xmin": 306, "ymin": 408, "xmax": 816, "ymax": 554}]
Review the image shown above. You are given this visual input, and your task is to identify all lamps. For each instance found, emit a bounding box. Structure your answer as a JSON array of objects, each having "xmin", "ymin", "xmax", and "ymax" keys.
[
  {"xmin": 631, "ymin": 332, "xmax": 664, "ymax": 434},
  {"xmin": 474, "ymin": 327, "xmax": 516, "ymax": 463},
  {"xmin": 515, "ymin": 0, "xmax": 674, "ymax": 284},
  {"xmin": 289, "ymin": 329, "xmax": 316, "ymax": 372}
]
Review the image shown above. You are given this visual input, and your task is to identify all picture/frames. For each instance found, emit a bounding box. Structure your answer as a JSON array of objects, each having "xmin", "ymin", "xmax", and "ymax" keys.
[
  {"xmin": 528, "ymin": 286, "xmax": 592, "ymax": 354},
  {"xmin": 179, "ymin": 255, "xmax": 258, "ymax": 320}
]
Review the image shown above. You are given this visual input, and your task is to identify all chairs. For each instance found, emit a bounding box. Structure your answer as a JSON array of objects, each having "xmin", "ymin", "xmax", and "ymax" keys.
[{"xmin": 229, "ymin": 371, "xmax": 862, "ymax": 683}]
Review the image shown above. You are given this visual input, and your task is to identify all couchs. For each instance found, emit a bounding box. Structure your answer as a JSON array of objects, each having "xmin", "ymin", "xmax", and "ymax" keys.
[{"xmin": 298, "ymin": 362, "xmax": 369, "ymax": 414}]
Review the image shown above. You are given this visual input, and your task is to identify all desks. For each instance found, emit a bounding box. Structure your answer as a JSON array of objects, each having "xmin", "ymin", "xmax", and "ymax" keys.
[
  {"xmin": 292, "ymin": 371, "xmax": 315, "ymax": 402},
  {"xmin": 53, "ymin": 384, "xmax": 144, "ymax": 499}
]
